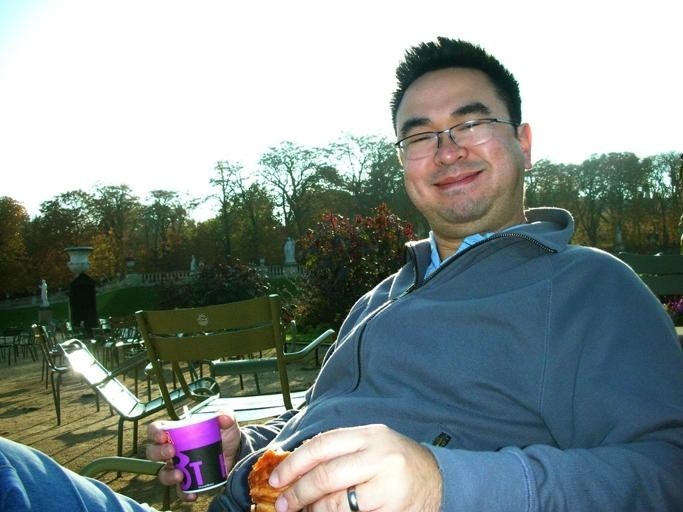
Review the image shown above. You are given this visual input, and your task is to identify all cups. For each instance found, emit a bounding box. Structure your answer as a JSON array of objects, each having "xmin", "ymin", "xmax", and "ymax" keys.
[{"xmin": 163, "ymin": 411, "xmax": 229, "ymax": 495}]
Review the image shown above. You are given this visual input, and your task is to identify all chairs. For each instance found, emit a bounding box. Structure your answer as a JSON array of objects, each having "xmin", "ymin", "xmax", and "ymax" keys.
[
  {"xmin": 100, "ymin": 315, "xmax": 147, "ymax": 380},
  {"xmin": 134, "ymin": 294, "xmax": 335, "ymax": 421},
  {"xmin": 56, "ymin": 319, "xmax": 100, "ymax": 425},
  {"xmin": 0, "ymin": 314, "xmax": 150, "ymax": 426},
  {"xmin": 57, "ymin": 294, "xmax": 336, "ymax": 512},
  {"xmin": 58, "ymin": 338, "xmax": 222, "ymax": 478}
]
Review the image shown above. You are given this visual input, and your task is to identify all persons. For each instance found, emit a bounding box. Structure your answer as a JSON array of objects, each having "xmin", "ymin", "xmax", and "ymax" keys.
[
  {"xmin": 39, "ymin": 279, "xmax": 49, "ymax": 305},
  {"xmin": 0, "ymin": 38, "xmax": 683, "ymax": 512}
]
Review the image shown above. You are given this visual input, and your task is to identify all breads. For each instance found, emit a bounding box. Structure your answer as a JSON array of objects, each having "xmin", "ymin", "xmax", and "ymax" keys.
[{"xmin": 247, "ymin": 448, "xmax": 304, "ymax": 512}]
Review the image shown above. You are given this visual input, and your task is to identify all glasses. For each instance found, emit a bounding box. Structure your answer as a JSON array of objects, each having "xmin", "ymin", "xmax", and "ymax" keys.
[{"xmin": 394, "ymin": 118, "xmax": 519, "ymax": 161}]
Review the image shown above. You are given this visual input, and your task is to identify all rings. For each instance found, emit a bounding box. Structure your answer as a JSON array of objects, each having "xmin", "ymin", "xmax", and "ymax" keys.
[{"xmin": 347, "ymin": 486, "xmax": 359, "ymax": 512}]
